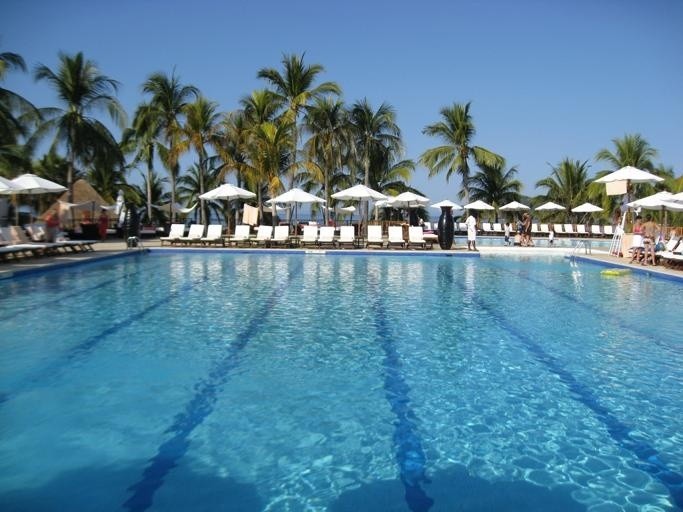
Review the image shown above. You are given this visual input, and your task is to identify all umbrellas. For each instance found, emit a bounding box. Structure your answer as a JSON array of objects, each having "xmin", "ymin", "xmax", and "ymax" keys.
[
  {"xmin": 0, "ymin": 175, "xmax": 20, "ymax": 193},
  {"xmin": 2, "ymin": 172, "xmax": 69, "ymax": 194},
  {"xmin": 198, "ymin": 182, "xmax": 257, "ymax": 239},
  {"xmin": 620, "ymin": 187, "xmax": 683, "ymax": 242},
  {"xmin": 592, "ymin": 164, "xmax": 665, "ymax": 216}
]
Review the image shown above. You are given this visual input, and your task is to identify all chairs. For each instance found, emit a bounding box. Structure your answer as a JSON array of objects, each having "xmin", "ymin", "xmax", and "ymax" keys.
[
  {"xmin": 626, "ymin": 233, "xmax": 683, "ymax": 267},
  {"xmin": 0, "ymin": 220, "xmax": 99, "ymax": 264},
  {"xmin": 158, "ymin": 220, "xmax": 438, "ymax": 250},
  {"xmin": 453, "ymin": 220, "xmax": 614, "ymax": 237}
]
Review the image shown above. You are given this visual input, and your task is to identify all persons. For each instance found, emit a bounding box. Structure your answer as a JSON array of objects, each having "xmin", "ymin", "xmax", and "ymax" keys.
[
  {"xmin": 465, "ymin": 212, "xmax": 478, "ymax": 251},
  {"xmin": 42, "ymin": 208, "xmax": 60, "ymax": 255},
  {"xmin": 97, "ymin": 208, "xmax": 108, "ymax": 242},
  {"xmin": 629, "ymin": 214, "xmax": 675, "ymax": 265},
  {"xmin": 501, "ymin": 212, "xmax": 534, "ymax": 247},
  {"xmin": 546, "ymin": 221, "xmax": 555, "ymax": 241}
]
